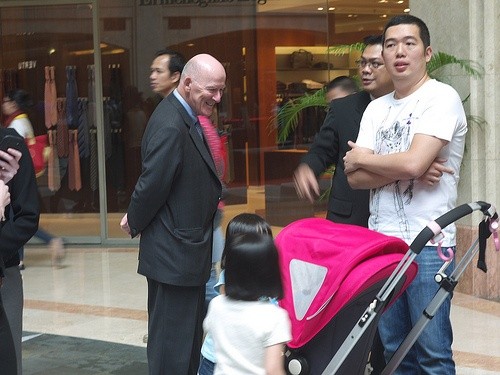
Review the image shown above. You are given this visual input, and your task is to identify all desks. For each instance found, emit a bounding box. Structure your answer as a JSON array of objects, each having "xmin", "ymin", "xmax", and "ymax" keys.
[{"xmin": 264, "ymin": 150, "xmax": 334, "ymax": 226}]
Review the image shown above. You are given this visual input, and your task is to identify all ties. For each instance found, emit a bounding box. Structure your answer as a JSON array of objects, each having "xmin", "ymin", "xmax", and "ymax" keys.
[{"xmin": 44, "ymin": 63, "xmax": 125, "ymax": 193}]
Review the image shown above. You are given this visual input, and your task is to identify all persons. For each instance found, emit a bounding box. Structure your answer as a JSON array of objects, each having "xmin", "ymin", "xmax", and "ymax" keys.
[
  {"xmin": 202, "ymin": 233, "xmax": 293, "ymax": 375},
  {"xmin": 293, "ymin": 35, "xmax": 396, "ymax": 375},
  {"xmin": 343, "ymin": 15, "xmax": 468, "ymax": 375},
  {"xmin": 196, "ymin": 212, "xmax": 275, "ymax": 375},
  {"xmin": 0, "ymin": 88, "xmax": 67, "ymax": 374},
  {"xmin": 120, "ymin": 53, "xmax": 226, "ymax": 375},
  {"xmin": 150, "ymin": 53, "xmax": 216, "ymax": 374},
  {"xmin": 326, "ymin": 75, "xmax": 360, "ymax": 103}
]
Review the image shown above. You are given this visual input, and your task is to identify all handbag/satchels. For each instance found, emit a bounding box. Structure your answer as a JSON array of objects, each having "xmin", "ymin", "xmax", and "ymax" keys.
[
  {"xmin": 312, "ymin": 61, "xmax": 334, "ymax": 69},
  {"xmin": 302, "ymin": 79, "xmax": 325, "ymax": 90},
  {"xmin": 288, "ymin": 82, "xmax": 307, "ymax": 94},
  {"xmin": 290, "ymin": 49, "xmax": 314, "ymax": 70},
  {"xmin": 276, "ymin": 81, "xmax": 286, "ymax": 93},
  {"xmin": 22, "ymin": 134, "xmax": 49, "ymax": 178}
]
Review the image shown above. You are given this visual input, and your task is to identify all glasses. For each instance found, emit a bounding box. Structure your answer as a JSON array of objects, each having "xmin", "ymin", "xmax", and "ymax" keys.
[{"xmin": 358, "ymin": 58, "xmax": 384, "ymax": 71}]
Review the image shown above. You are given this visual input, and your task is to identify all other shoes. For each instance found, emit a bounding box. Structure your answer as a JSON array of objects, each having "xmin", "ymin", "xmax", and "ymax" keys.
[
  {"xmin": 19, "ymin": 264, "xmax": 24, "ymax": 270},
  {"xmin": 51, "ymin": 236, "xmax": 65, "ymax": 265}
]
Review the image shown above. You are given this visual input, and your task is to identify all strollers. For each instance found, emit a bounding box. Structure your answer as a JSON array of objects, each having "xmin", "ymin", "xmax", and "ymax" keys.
[{"xmin": 274, "ymin": 200, "xmax": 500, "ymax": 375}]
